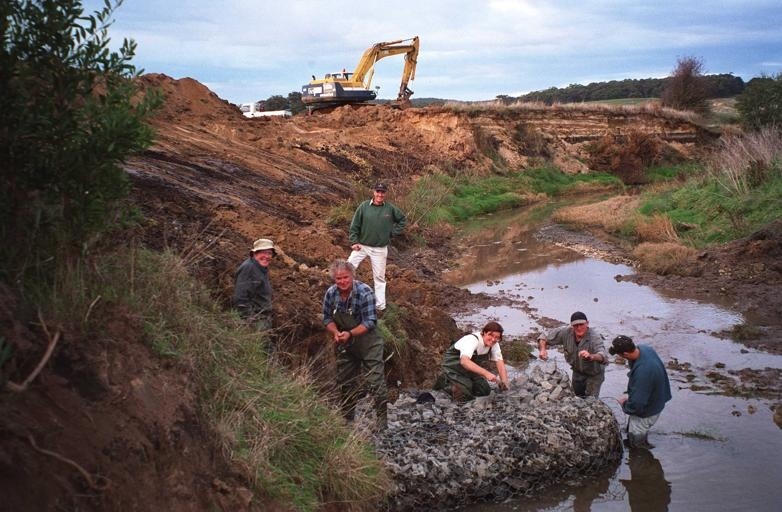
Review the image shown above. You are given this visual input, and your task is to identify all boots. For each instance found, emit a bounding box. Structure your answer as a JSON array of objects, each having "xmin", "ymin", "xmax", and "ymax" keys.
[
  {"xmin": 341, "ymin": 391, "xmax": 355, "ymax": 420},
  {"xmin": 373, "ymin": 397, "xmax": 389, "ymax": 431}
]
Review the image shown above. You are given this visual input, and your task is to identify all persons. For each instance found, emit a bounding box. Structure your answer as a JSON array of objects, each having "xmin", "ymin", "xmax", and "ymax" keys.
[
  {"xmin": 536, "ymin": 311, "xmax": 609, "ymax": 400},
  {"xmin": 232, "ymin": 238, "xmax": 277, "ymax": 354},
  {"xmin": 323, "ymin": 259, "xmax": 389, "ymax": 432},
  {"xmin": 609, "ymin": 335, "xmax": 671, "ymax": 449},
  {"xmin": 617, "ymin": 449, "xmax": 672, "ymax": 511},
  {"xmin": 433, "ymin": 322, "xmax": 510, "ymax": 403},
  {"xmin": 346, "ymin": 182, "xmax": 407, "ymax": 318}
]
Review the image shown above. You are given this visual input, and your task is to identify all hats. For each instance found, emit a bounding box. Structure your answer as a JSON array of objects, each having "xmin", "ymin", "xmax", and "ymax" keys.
[
  {"xmin": 249, "ymin": 239, "xmax": 278, "ymax": 258},
  {"xmin": 375, "ymin": 183, "xmax": 387, "ymax": 192},
  {"xmin": 609, "ymin": 335, "xmax": 633, "ymax": 356},
  {"xmin": 570, "ymin": 311, "xmax": 587, "ymax": 326}
]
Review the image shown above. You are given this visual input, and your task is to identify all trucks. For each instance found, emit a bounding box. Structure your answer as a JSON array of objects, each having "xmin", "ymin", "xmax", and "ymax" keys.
[{"xmin": 239, "ymin": 102, "xmax": 292, "ymax": 118}]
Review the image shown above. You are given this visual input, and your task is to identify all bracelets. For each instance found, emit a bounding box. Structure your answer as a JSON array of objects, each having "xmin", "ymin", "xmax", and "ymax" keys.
[{"xmin": 348, "ymin": 331, "xmax": 353, "ymax": 336}]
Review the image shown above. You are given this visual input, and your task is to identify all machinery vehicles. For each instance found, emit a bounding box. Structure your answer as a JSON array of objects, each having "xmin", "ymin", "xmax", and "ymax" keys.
[{"xmin": 300, "ymin": 35, "xmax": 419, "ymax": 116}]
